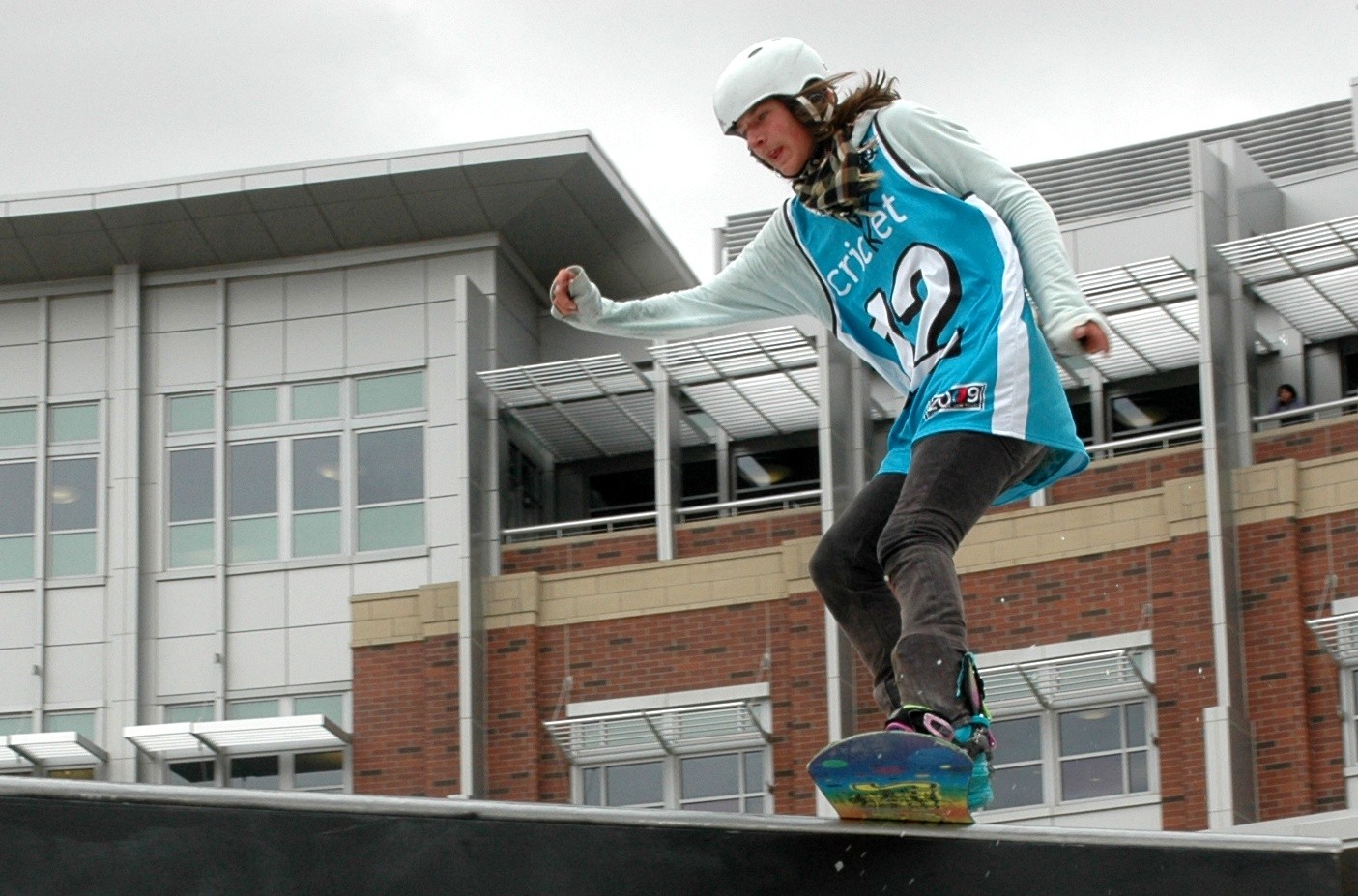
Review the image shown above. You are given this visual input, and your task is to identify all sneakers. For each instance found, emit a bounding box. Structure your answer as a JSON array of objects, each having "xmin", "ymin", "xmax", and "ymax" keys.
[{"xmin": 888, "ymin": 711, "xmax": 993, "ymax": 810}]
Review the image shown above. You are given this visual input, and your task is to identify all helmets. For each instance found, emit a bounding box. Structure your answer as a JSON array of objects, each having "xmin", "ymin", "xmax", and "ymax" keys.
[{"xmin": 714, "ymin": 35, "xmax": 828, "ymax": 135}]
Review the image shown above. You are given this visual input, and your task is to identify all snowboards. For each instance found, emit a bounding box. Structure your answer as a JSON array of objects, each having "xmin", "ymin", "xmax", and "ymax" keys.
[{"xmin": 805, "ymin": 728, "xmax": 976, "ymax": 824}]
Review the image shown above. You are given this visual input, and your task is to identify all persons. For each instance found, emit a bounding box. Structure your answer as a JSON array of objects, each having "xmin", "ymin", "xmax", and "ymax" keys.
[
  {"xmin": 1268, "ymin": 384, "xmax": 1314, "ymax": 427},
  {"xmin": 548, "ymin": 38, "xmax": 1112, "ymax": 811}
]
